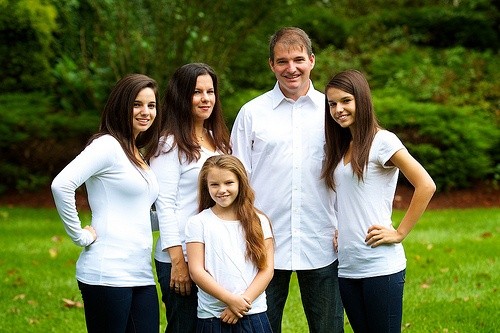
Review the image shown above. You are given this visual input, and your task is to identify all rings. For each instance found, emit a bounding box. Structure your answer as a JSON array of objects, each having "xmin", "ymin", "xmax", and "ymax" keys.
[{"xmin": 175, "ymin": 286, "xmax": 180, "ymax": 289}]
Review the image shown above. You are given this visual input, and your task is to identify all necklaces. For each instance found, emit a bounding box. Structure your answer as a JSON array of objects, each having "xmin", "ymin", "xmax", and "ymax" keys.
[{"xmin": 194, "ymin": 130, "xmax": 206, "ymax": 141}]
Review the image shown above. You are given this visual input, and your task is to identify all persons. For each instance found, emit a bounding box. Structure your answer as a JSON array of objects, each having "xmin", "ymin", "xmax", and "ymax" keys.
[
  {"xmin": 229, "ymin": 27, "xmax": 344, "ymax": 333},
  {"xmin": 186, "ymin": 154, "xmax": 274, "ymax": 333},
  {"xmin": 51, "ymin": 75, "xmax": 160, "ymax": 333},
  {"xmin": 147, "ymin": 62, "xmax": 233, "ymax": 333},
  {"xmin": 319, "ymin": 70, "xmax": 437, "ymax": 332}
]
